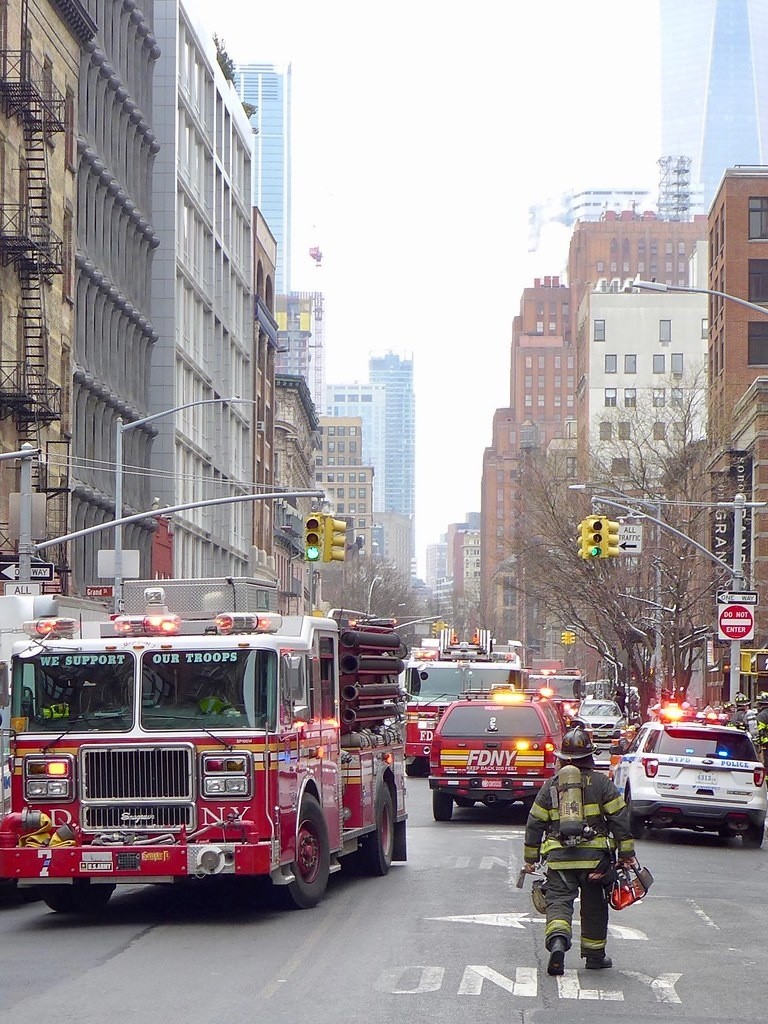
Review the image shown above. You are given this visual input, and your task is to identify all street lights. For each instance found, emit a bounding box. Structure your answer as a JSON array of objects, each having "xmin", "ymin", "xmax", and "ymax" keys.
[
  {"xmin": 569, "ymin": 485, "xmax": 663, "ymax": 702},
  {"xmin": 116, "ymin": 399, "xmax": 258, "ymax": 613}
]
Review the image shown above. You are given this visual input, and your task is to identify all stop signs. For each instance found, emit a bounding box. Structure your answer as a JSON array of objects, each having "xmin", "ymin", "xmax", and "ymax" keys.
[{"xmin": 720, "ymin": 606, "xmax": 753, "ymax": 639}]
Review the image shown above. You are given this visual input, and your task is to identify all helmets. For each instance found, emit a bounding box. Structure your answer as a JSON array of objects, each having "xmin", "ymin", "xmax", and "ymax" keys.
[
  {"xmin": 724, "ymin": 702, "xmax": 734, "ymax": 709},
  {"xmin": 757, "ymin": 692, "xmax": 768, "ymax": 703},
  {"xmin": 682, "ymin": 702, "xmax": 690, "ymax": 710},
  {"xmin": 735, "ymin": 694, "xmax": 751, "ymax": 706},
  {"xmin": 553, "ymin": 726, "xmax": 597, "ymax": 760}
]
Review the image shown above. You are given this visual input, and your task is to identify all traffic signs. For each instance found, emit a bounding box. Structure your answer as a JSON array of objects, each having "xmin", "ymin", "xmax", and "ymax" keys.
[
  {"xmin": 617, "ymin": 524, "xmax": 642, "ymax": 554},
  {"xmin": 716, "ymin": 591, "xmax": 759, "ymax": 606}
]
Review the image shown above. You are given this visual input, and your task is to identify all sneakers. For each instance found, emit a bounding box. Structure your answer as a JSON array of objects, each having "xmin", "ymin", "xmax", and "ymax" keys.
[
  {"xmin": 586, "ymin": 957, "xmax": 613, "ymax": 968},
  {"xmin": 547, "ymin": 937, "xmax": 564, "ymax": 975}
]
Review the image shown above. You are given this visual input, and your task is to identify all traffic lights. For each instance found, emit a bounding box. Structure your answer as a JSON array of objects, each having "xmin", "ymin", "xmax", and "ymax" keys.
[
  {"xmin": 302, "ymin": 514, "xmax": 325, "ymax": 563},
  {"xmin": 578, "ymin": 517, "xmax": 619, "ymax": 559},
  {"xmin": 561, "ymin": 630, "xmax": 576, "ymax": 644},
  {"xmin": 756, "ymin": 653, "xmax": 768, "ymax": 671},
  {"xmin": 739, "ymin": 653, "xmax": 751, "ymax": 672},
  {"xmin": 323, "ymin": 517, "xmax": 348, "ymax": 563},
  {"xmin": 431, "ymin": 620, "xmax": 448, "ymax": 634}
]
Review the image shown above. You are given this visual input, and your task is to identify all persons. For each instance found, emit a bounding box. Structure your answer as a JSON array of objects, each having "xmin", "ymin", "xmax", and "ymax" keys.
[
  {"xmin": 629, "ymin": 690, "xmax": 638, "ymax": 713},
  {"xmin": 754, "ymin": 691, "xmax": 768, "ymax": 792},
  {"xmin": 723, "ymin": 702, "xmax": 736, "ymax": 713},
  {"xmin": 617, "ymin": 694, "xmax": 624, "ymax": 713},
  {"xmin": 726, "ymin": 693, "xmax": 751, "ymax": 732},
  {"xmin": 525, "ymin": 729, "xmax": 637, "ymax": 976}
]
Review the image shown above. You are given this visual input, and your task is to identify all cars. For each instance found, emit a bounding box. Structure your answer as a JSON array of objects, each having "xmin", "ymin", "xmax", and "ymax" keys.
[
  {"xmin": 607, "ymin": 710, "xmax": 767, "ymax": 848},
  {"xmin": 577, "ymin": 699, "xmax": 630, "ymax": 739}
]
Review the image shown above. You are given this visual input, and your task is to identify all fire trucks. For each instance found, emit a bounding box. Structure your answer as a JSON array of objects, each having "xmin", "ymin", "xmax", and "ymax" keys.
[
  {"xmin": 399, "ymin": 627, "xmax": 523, "ymax": 778},
  {"xmin": 528, "ymin": 659, "xmax": 585, "ymax": 721},
  {"xmin": 1, "ymin": 578, "xmax": 410, "ymax": 913}
]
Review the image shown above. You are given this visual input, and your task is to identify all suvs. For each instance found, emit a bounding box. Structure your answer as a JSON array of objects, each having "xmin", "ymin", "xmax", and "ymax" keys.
[{"xmin": 428, "ymin": 685, "xmax": 568, "ymax": 821}]
